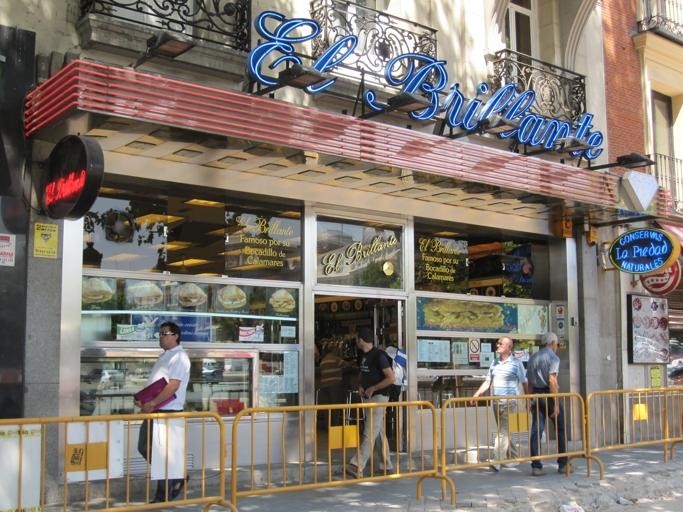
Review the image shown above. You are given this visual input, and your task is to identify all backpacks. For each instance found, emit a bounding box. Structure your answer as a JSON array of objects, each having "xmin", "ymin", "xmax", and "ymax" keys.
[{"xmin": 375, "ymin": 345, "xmax": 406, "ymax": 401}]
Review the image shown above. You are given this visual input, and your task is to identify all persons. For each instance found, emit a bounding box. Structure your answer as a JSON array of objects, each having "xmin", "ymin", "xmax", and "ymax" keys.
[
  {"xmin": 470, "ymin": 337, "xmax": 528, "ymax": 472},
  {"xmin": 525, "ymin": 332, "xmax": 575, "ymax": 476},
  {"xmin": 319, "ymin": 328, "xmax": 405, "ymax": 479},
  {"xmin": 134, "ymin": 322, "xmax": 191, "ymax": 504}
]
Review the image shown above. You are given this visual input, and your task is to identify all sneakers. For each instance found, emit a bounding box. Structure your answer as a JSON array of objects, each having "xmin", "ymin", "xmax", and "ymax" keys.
[
  {"xmin": 486, "ymin": 459, "xmax": 499, "ymax": 471},
  {"xmin": 504, "ymin": 462, "xmax": 520, "ymax": 467},
  {"xmin": 558, "ymin": 465, "xmax": 576, "ymax": 473},
  {"xmin": 151, "ymin": 476, "xmax": 189, "ymax": 503},
  {"xmin": 342, "ymin": 463, "xmax": 362, "ymax": 479},
  {"xmin": 369, "ymin": 470, "xmax": 391, "ymax": 476},
  {"xmin": 533, "ymin": 467, "xmax": 547, "ymax": 476}
]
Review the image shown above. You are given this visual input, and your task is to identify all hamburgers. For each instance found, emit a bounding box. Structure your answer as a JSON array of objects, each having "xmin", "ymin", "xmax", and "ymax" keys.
[
  {"xmin": 175, "ymin": 282, "xmax": 208, "ymax": 307},
  {"xmin": 127, "ymin": 285, "xmax": 164, "ymax": 306},
  {"xmin": 83, "ymin": 278, "xmax": 114, "ymax": 305},
  {"xmin": 269, "ymin": 290, "xmax": 295, "ymax": 312},
  {"xmin": 422, "ymin": 300, "xmax": 504, "ymax": 330},
  {"xmin": 216, "ymin": 285, "xmax": 247, "ymax": 310}
]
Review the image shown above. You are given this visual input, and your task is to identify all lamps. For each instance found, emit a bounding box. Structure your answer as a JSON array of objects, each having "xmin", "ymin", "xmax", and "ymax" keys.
[
  {"xmin": 521, "ymin": 130, "xmax": 657, "ymax": 174},
  {"xmin": 356, "ymin": 92, "xmax": 523, "ymax": 144},
  {"xmin": 128, "ymin": 26, "xmax": 329, "ymax": 100}
]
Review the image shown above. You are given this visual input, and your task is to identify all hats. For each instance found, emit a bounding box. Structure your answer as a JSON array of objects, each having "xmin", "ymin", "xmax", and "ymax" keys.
[{"xmin": 358, "ymin": 327, "xmax": 374, "ymax": 341}]
